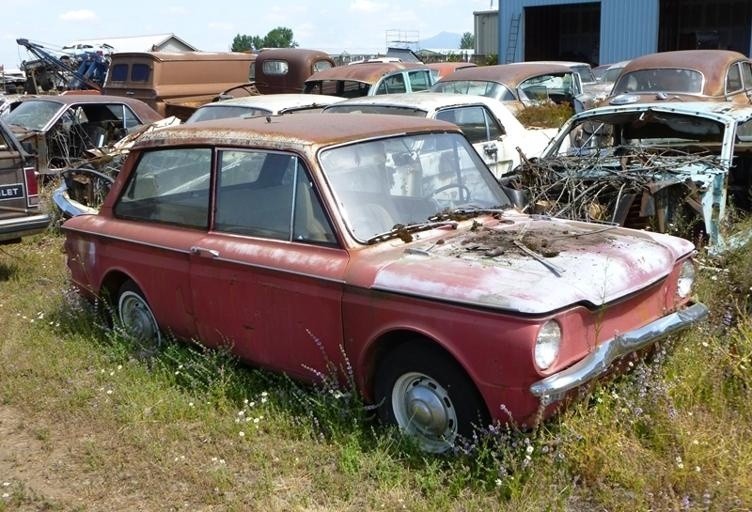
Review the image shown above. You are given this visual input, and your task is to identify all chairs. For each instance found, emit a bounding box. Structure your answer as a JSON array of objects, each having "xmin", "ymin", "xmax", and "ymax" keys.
[{"xmin": 277, "ymin": 181, "xmax": 393, "ymax": 249}]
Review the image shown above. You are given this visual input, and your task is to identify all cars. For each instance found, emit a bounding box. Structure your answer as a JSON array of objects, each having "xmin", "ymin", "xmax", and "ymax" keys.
[
  {"xmin": 0, "ymin": 119, "xmax": 51, "ymax": 246},
  {"xmin": 53, "ymin": 94, "xmax": 350, "ymax": 231},
  {"xmin": 2, "ymin": 95, "xmax": 167, "ymax": 184},
  {"xmin": 536, "ymin": 47, "xmax": 752, "ymax": 199},
  {"xmin": 61, "ymin": 111, "xmax": 708, "ymax": 466},
  {"xmin": 426, "ymin": 63, "xmax": 598, "ymax": 114},
  {"xmin": 498, "ymin": 101, "xmax": 751, "ymax": 263},
  {"xmin": 319, "ymin": 91, "xmax": 570, "ymax": 209},
  {"xmin": 1, "ymin": 39, "xmax": 751, "ymax": 118}
]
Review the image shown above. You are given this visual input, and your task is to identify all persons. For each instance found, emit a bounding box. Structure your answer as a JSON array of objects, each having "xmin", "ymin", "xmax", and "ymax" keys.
[{"xmin": 51, "ymin": 54, "xmax": 80, "ymax": 90}]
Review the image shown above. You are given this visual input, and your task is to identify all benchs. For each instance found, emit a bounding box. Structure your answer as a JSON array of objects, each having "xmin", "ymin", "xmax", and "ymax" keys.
[{"xmin": 148, "ymin": 182, "xmax": 311, "ymax": 235}]
